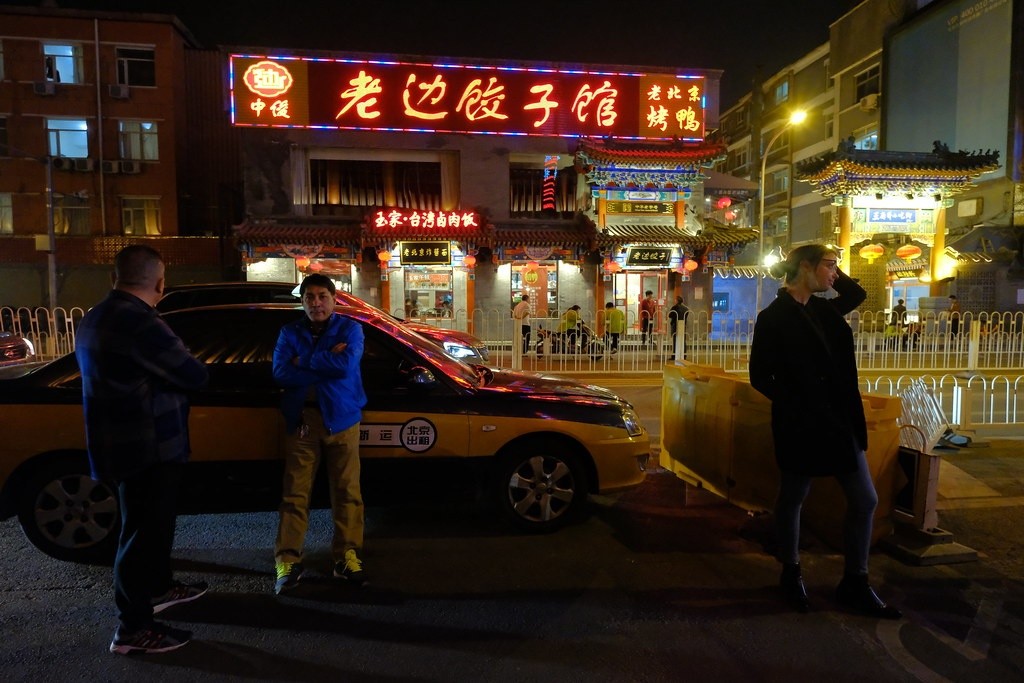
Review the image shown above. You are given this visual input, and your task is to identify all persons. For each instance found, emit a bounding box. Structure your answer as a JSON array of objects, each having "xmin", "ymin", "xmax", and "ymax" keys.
[
  {"xmin": 641, "ymin": 291, "xmax": 655, "ymax": 344},
  {"xmin": 557, "ymin": 305, "xmax": 587, "ymax": 350},
  {"xmin": 405, "ymin": 299, "xmax": 417, "ymax": 317},
  {"xmin": 944, "ymin": 295, "xmax": 960, "ymax": 340},
  {"xmin": 73, "ymin": 245, "xmax": 210, "ymax": 652},
  {"xmin": 602, "ymin": 302, "xmax": 625, "ymax": 354},
  {"xmin": 884, "ymin": 299, "xmax": 920, "ymax": 351},
  {"xmin": 442, "ymin": 301, "xmax": 450, "ymax": 317},
  {"xmin": 513, "ymin": 295, "xmax": 531, "ymax": 357},
  {"xmin": 750, "ymin": 244, "xmax": 901, "ymax": 621},
  {"xmin": 272, "ymin": 274, "xmax": 366, "ymax": 595},
  {"xmin": 668, "ymin": 296, "xmax": 689, "ymax": 360}
]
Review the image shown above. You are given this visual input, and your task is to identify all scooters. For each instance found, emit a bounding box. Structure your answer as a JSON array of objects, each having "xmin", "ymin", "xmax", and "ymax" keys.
[{"xmin": 533, "ymin": 318, "xmax": 607, "ymax": 361}]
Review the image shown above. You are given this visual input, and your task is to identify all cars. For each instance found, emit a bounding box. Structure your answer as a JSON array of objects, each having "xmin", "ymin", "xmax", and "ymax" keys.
[
  {"xmin": 151, "ymin": 283, "xmax": 490, "ymax": 374},
  {"xmin": 0, "ymin": 299, "xmax": 655, "ymax": 563},
  {"xmin": 0, "ymin": 331, "xmax": 30, "ymax": 364}
]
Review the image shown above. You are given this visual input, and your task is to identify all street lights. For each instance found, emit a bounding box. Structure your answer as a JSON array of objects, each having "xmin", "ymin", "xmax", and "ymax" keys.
[{"xmin": 755, "ymin": 108, "xmax": 809, "ymax": 318}]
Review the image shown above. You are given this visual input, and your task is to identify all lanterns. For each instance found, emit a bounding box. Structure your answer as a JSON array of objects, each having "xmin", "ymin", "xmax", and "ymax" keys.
[
  {"xmin": 609, "ymin": 261, "xmax": 619, "ymax": 273},
  {"xmin": 859, "ymin": 244, "xmax": 884, "ymax": 264},
  {"xmin": 378, "ymin": 251, "xmax": 391, "ymax": 267},
  {"xmin": 718, "ymin": 197, "xmax": 738, "ymax": 220},
  {"xmin": 297, "ymin": 256, "xmax": 322, "ymax": 274},
  {"xmin": 684, "ymin": 260, "xmax": 698, "ymax": 272},
  {"xmin": 896, "ymin": 245, "xmax": 922, "ymax": 264},
  {"xmin": 527, "ymin": 261, "xmax": 539, "ymax": 272},
  {"xmin": 464, "ymin": 256, "xmax": 476, "ymax": 270}
]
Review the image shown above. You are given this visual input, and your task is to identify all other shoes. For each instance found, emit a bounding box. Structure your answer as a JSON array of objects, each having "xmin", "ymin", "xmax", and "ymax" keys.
[
  {"xmin": 837, "ymin": 568, "xmax": 903, "ymax": 618},
  {"xmin": 780, "ymin": 565, "xmax": 808, "ymax": 612}
]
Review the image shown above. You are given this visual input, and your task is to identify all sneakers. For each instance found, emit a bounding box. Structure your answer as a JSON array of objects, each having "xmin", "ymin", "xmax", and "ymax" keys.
[
  {"xmin": 332, "ymin": 550, "xmax": 370, "ymax": 588},
  {"xmin": 109, "ymin": 624, "xmax": 194, "ymax": 656},
  {"xmin": 147, "ymin": 580, "xmax": 209, "ymax": 613},
  {"xmin": 274, "ymin": 560, "xmax": 307, "ymax": 594}
]
